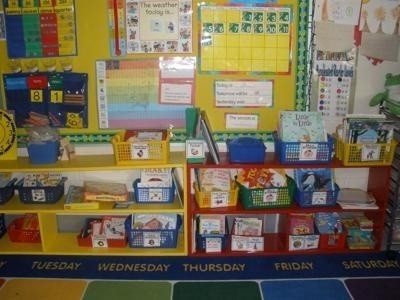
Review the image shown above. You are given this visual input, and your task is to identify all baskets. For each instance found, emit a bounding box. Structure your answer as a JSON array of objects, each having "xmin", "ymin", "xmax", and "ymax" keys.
[
  {"xmin": 285, "ymin": 175, "xmax": 340, "ymax": 207},
  {"xmin": 112, "ymin": 132, "xmax": 170, "ymax": 164},
  {"xmin": 275, "ymin": 134, "xmax": 333, "ymax": 165},
  {"xmin": 332, "ymin": 131, "xmax": 398, "ymax": 166},
  {"xmin": 197, "ymin": 233, "xmax": 225, "ymax": 250},
  {"xmin": 193, "ymin": 180, "xmax": 239, "ymax": 209},
  {"xmin": 125, "ymin": 214, "xmax": 182, "ymax": 248},
  {"xmin": 16, "ymin": 175, "xmax": 67, "ymax": 203},
  {"xmin": 8, "ymin": 217, "xmax": 41, "ymax": 243},
  {"xmin": 0, "ymin": 177, "xmax": 17, "ymax": 204},
  {"xmin": 235, "ymin": 173, "xmax": 295, "ymax": 208},
  {"xmin": 133, "ymin": 178, "xmax": 175, "ymax": 203}
]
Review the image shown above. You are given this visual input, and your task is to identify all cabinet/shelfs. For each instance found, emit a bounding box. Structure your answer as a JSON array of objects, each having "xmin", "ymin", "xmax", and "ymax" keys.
[
  {"xmin": 186, "ymin": 98, "xmax": 400, "ymax": 257},
  {"xmin": 0, "ymin": 99, "xmax": 187, "ymax": 258}
]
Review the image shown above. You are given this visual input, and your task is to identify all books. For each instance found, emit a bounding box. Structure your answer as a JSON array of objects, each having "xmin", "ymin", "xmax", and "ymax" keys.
[{"xmin": 1, "ymin": 111, "xmax": 395, "ymax": 247}]
